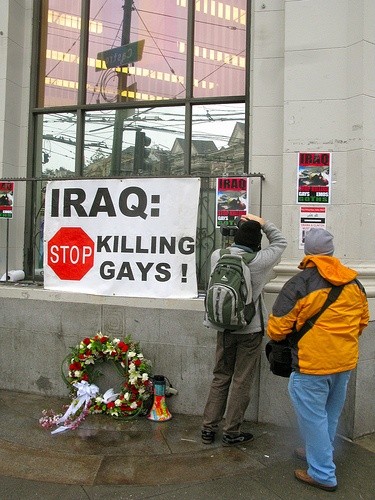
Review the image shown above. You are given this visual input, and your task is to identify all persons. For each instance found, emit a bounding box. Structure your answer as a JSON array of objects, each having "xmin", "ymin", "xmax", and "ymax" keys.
[
  {"xmin": 199, "ymin": 215, "xmax": 288, "ymax": 446},
  {"xmin": 267, "ymin": 229, "xmax": 369, "ymax": 491}
]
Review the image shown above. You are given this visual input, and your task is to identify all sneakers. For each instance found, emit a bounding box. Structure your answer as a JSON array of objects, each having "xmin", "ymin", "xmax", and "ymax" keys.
[
  {"xmin": 201, "ymin": 429, "xmax": 215, "ymax": 444},
  {"xmin": 222, "ymin": 431, "xmax": 254, "ymax": 447}
]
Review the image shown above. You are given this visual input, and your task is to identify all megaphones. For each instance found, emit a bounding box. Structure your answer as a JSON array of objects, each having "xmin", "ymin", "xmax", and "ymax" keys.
[{"xmin": 146, "ymin": 375, "xmax": 178, "ymax": 421}]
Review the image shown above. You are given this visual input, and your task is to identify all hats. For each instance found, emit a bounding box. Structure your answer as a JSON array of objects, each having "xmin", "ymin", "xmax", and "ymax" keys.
[
  {"xmin": 234, "ymin": 221, "xmax": 263, "ymax": 252},
  {"xmin": 304, "ymin": 227, "xmax": 334, "ymax": 257}
]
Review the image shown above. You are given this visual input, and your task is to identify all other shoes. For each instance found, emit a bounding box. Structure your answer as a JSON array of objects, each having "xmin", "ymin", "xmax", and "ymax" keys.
[
  {"xmin": 295, "ymin": 448, "xmax": 307, "ymax": 461},
  {"xmin": 294, "ymin": 469, "xmax": 338, "ymax": 492}
]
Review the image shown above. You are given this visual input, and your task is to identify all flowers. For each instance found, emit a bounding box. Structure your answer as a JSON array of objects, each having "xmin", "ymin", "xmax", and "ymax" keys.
[{"xmin": 38, "ymin": 331, "xmax": 154, "ymax": 434}]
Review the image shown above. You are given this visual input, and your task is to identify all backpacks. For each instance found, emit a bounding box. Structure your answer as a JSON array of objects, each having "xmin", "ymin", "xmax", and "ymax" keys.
[{"xmin": 205, "ymin": 249, "xmax": 261, "ymax": 330}]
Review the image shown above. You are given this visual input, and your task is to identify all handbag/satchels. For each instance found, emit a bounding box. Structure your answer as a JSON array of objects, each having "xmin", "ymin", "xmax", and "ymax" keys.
[{"xmin": 265, "ymin": 334, "xmax": 293, "ymax": 378}]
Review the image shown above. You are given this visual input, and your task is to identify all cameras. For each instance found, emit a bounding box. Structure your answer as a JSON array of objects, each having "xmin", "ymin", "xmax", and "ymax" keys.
[{"xmin": 220, "ymin": 218, "xmax": 250, "ymax": 236}]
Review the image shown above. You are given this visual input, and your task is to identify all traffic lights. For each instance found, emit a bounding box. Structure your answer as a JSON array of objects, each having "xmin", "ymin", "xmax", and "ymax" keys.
[{"xmin": 136, "ymin": 132, "xmax": 151, "ymax": 170}]
